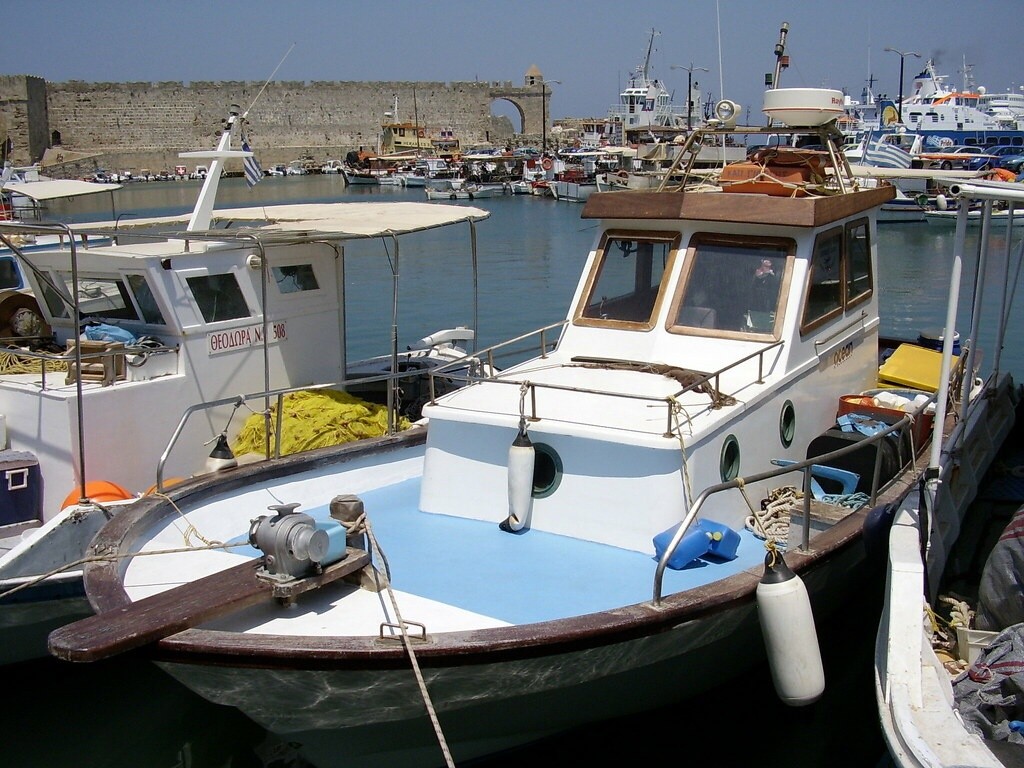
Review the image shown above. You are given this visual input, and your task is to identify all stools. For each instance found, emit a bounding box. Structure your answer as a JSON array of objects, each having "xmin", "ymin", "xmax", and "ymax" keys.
[{"xmin": 64, "ymin": 338, "xmax": 127, "ymax": 387}]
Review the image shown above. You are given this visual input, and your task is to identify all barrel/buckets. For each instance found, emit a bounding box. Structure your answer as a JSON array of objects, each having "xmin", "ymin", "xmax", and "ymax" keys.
[
  {"xmin": 652, "ymin": 522, "xmax": 713, "ymax": 570},
  {"xmin": 700, "ymin": 518, "xmax": 741, "ymax": 559},
  {"xmin": 916, "ymin": 328, "xmax": 961, "ymax": 356}
]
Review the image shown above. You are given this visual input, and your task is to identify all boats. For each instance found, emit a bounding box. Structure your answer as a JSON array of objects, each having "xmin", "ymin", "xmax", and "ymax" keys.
[
  {"xmin": 869, "ymin": 178, "xmax": 1024, "ymax": 768},
  {"xmin": 42, "ymin": 16, "xmax": 988, "ymax": 734},
  {"xmin": 0, "ymin": 104, "xmax": 502, "ymax": 632},
  {"xmin": 1, "ymin": 19, "xmax": 1024, "ymax": 228}
]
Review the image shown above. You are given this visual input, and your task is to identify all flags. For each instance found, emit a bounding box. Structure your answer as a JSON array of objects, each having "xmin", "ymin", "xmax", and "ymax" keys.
[
  {"xmin": 862, "ymin": 129, "xmax": 913, "ymax": 170},
  {"xmin": 240, "ymin": 130, "xmax": 267, "ymax": 190}
]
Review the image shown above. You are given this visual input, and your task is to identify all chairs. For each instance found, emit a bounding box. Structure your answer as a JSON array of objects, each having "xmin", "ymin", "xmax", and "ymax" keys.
[{"xmin": 677, "ymin": 306, "xmax": 719, "ymax": 329}]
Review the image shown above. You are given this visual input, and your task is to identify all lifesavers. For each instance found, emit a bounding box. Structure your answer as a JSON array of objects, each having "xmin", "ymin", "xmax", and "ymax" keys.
[
  {"xmin": 418, "ymin": 130, "xmax": 424, "ymax": 138},
  {"xmin": 459, "ymin": 165, "xmax": 469, "ymax": 176},
  {"xmin": 454, "ymin": 153, "xmax": 462, "ymax": 162},
  {"xmin": 542, "ymin": 158, "xmax": 552, "ymax": 170},
  {"xmin": 616, "ymin": 170, "xmax": 629, "ymax": 184},
  {"xmin": 444, "ymin": 155, "xmax": 453, "ymax": 163}
]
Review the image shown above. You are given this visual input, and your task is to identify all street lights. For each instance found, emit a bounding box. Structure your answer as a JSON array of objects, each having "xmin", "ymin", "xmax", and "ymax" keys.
[
  {"xmin": 669, "ymin": 61, "xmax": 708, "ymax": 130},
  {"xmin": 881, "ymin": 48, "xmax": 922, "ymax": 124},
  {"xmin": 525, "ymin": 79, "xmax": 562, "ymax": 148}
]
